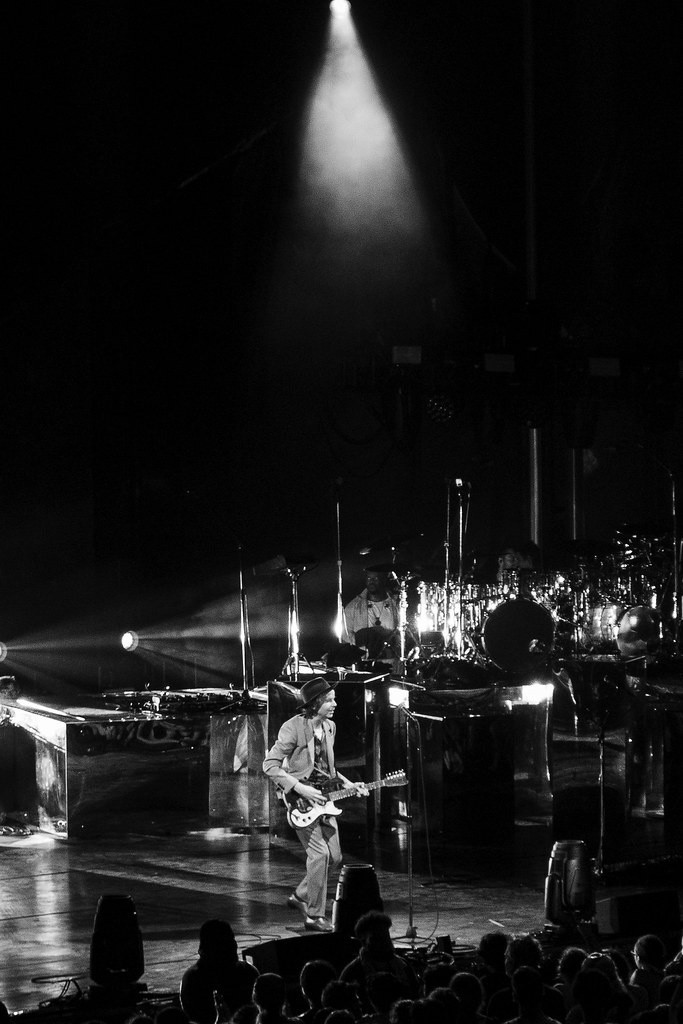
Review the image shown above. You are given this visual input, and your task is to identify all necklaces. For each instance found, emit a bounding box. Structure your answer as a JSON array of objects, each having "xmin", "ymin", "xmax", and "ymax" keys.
[{"xmin": 367, "ymin": 598, "xmax": 386, "ymax": 626}]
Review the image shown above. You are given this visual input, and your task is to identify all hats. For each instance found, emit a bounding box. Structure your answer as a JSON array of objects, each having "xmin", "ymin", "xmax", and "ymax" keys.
[{"xmin": 295, "ymin": 676, "xmax": 338, "ymax": 710}]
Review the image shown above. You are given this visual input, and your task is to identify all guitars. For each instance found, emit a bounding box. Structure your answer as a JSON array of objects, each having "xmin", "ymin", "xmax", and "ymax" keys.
[{"xmin": 282, "ymin": 768, "xmax": 410, "ymax": 830}]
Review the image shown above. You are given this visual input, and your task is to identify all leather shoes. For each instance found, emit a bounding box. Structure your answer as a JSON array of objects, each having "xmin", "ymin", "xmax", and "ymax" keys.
[{"xmin": 288, "ymin": 893, "xmax": 334, "ymax": 933}]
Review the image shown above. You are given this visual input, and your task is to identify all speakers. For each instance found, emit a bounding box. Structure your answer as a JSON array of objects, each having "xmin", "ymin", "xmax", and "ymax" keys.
[
  {"xmin": 240, "ymin": 931, "xmax": 355, "ymax": 980},
  {"xmin": 331, "ymin": 864, "xmax": 384, "ymax": 931},
  {"xmin": 89, "ymin": 894, "xmax": 145, "ymax": 986},
  {"xmin": 597, "ymin": 889, "xmax": 680, "ymax": 936},
  {"xmin": 543, "ymin": 840, "xmax": 597, "ymax": 925}
]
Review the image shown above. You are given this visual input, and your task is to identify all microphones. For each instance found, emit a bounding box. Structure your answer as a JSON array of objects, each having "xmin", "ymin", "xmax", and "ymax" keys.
[{"xmin": 397, "ymin": 703, "xmax": 418, "ymax": 722}]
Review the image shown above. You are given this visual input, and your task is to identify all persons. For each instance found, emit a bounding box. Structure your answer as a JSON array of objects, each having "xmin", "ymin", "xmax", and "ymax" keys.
[
  {"xmin": 262, "ymin": 676, "xmax": 369, "ymax": 932},
  {"xmin": 343, "ymin": 568, "xmax": 402, "ymax": 646},
  {"xmin": 129, "ymin": 905, "xmax": 683, "ymax": 1024}
]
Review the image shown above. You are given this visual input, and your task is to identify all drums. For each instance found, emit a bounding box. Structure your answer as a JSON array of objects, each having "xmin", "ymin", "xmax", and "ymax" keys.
[{"xmin": 473, "ymin": 596, "xmax": 556, "ymax": 677}]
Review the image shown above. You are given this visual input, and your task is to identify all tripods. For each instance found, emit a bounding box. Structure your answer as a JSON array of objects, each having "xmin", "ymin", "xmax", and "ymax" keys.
[{"xmin": 391, "ymin": 716, "xmax": 436, "ymax": 949}]
